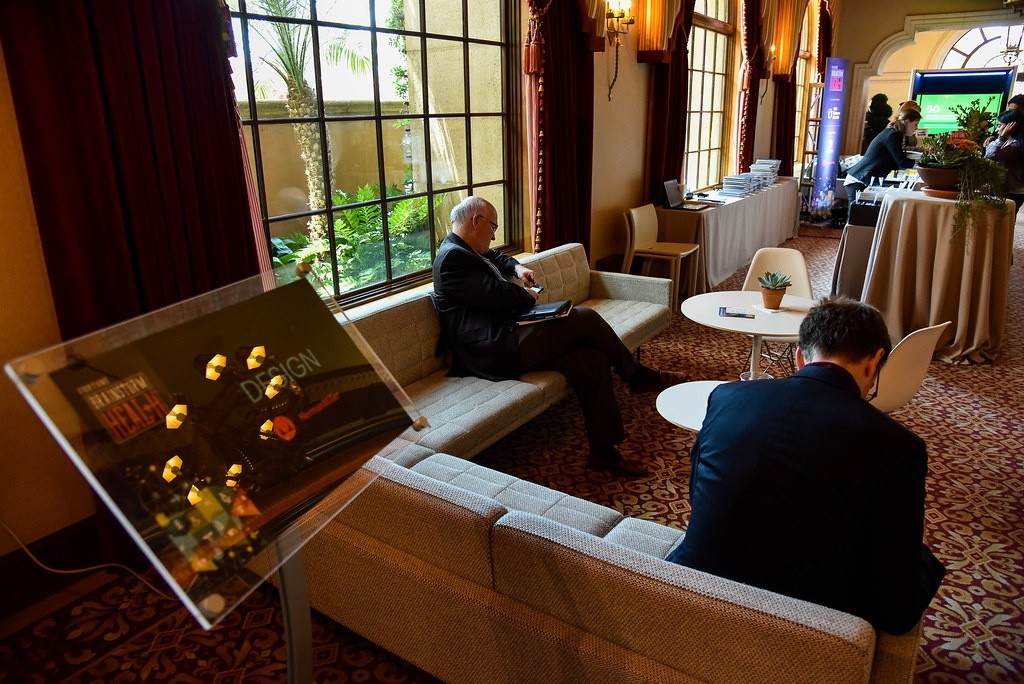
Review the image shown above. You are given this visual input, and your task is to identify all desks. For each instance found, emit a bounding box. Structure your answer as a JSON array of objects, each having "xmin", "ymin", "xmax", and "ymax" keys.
[
  {"xmin": 655, "ymin": 176, "xmax": 800, "ymax": 299},
  {"xmin": 804, "ymin": 176, "xmax": 849, "ymax": 200},
  {"xmin": 830, "ymin": 220, "xmax": 876, "ymax": 301},
  {"xmin": 655, "ymin": 290, "xmax": 821, "ymax": 435},
  {"xmin": 859, "ymin": 190, "xmax": 1016, "ymax": 366}
]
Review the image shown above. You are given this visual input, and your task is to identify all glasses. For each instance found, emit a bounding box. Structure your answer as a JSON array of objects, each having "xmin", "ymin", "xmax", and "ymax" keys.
[
  {"xmin": 866, "ymin": 368, "xmax": 880, "ymax": 402},
  {"xmin": 471, "ymin": 215, "xmax": 498, "ymax": 233}
]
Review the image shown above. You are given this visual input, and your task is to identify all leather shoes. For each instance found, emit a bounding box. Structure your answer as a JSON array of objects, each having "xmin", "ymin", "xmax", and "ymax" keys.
[
  {"xmin": 610, "ymin": 457, "xmax": 648, "ymax": 477},
  {"xmin": 651, "ymin": 369, "xmax": 687, "ymax": 393}
]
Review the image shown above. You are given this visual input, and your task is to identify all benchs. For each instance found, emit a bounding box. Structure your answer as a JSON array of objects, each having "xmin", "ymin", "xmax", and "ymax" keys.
[
  {"xmin": 268, "ymin": 452, "xmax": 928, "ymax": 684},
  {"xmin": 241, "ymin": 241, "xmax": 674, "ymax": 583}
]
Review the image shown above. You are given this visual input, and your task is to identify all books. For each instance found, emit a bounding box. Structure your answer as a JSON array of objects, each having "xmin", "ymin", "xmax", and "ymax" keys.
[{"xmin": 516, "ymin": 300, "xmax": 574, "ymax": 326}]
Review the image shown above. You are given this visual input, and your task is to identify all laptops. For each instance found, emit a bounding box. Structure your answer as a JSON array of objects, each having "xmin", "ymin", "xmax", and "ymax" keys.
[{"xmin": 665, "ymin": 179, "xmax": 709, "ymax": 210}]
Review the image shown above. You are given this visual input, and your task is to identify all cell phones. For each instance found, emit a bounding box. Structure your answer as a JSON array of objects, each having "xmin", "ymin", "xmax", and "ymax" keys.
[{"xmin": 531, "ymin": 285, "xmax": 544, "ymax": 294}]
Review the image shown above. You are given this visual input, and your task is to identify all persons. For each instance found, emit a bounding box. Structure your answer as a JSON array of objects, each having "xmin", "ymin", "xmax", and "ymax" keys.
[
  {"xmin": 984, "ymin": 92, "xmax": 1024, "ymax": 266},
  {"xmin": 842, "ymin": 94, "xmax": 924, "ymax": 218},
  {"xmin": 433, "ymin": 196, "xmax": 687, "ymax": 477},
  {"xmin": 666, "ymin": 296, "xmax": 945, "ymax": 636}
]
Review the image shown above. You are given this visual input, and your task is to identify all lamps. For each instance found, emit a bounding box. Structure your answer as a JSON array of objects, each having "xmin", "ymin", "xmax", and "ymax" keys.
[
  {"xmin": 604, "ymin": 0, "xmax": 635, "ymax": 102},
  {"xmin": 761, "ymin": 44, "xmax": 776, "ymax": 106}
]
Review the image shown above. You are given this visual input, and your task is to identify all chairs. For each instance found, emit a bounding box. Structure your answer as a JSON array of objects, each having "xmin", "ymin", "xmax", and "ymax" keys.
[
  {"xmin": 624, "ymin": 203, "xmax": 699, "ymax": 314},
  {"xmin": 867, "ymin": 321, "xmax": 953, "ymax": 416},
  {"xmin": 738, "ymin": 246, "xmax": 814, "ymax": 377},
  {"xmin": 620, "ymin": 211, "xmax": 694, "ymax": 312}
]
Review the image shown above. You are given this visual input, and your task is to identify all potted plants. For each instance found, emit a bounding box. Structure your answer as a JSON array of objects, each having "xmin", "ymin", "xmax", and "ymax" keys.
[
  {"xmin": 757, "ymin": 270, "xmax": 793, "ymax": 310},
  {"xmin": 915, "ymin": 132, "xmax": 1010, "ymax": 257}
]
